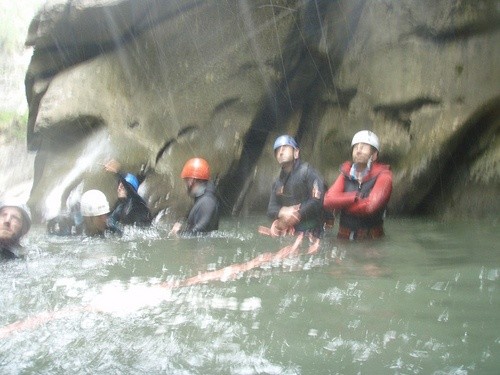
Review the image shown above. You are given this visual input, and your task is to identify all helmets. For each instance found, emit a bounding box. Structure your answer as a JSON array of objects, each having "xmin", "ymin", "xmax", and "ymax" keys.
[
  {"xmin": 273, "ymin": 135, "xmax": 298, "ymax": 156},
  {"xmin": 126, "ymin": 173, "xmax": 139, "ymax": 192},
  {"xmin": 0, "ymin": 197, "xmax": 32, "ymax": 234},
  {"xmin": 350, "ymin": 130, "xmax": 379, "ymax": 152},
  {"xmin": 80, "ymin": 189, "xmax": 110, "ymax": 217},
  {"xmin": 181, "ymin": 158, "xmax": 210, "ymax": 180}
]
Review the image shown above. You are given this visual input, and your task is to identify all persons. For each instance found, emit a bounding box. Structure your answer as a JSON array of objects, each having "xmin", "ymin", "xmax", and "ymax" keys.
[
  {"xmin": 0, "ymin": 195, "xmax": 32, "ymax": 260},
  {"xmin": 267, "ymin": 134, "xmax": 333, "ymax": 239},
  {"xmin": 95, "ymin": 158, "xmax": 152, "ymax": 228},
  {"xmin": 170, "ymin": 159, "xmax": 219, "ymax": 235},
  {"xmin": 323, "ymin": 130, "xmax": 393, "ymax": 240},
  {"xmin": 48, "ymin": 189, "xmax": 124, "ymax": 238}
]
